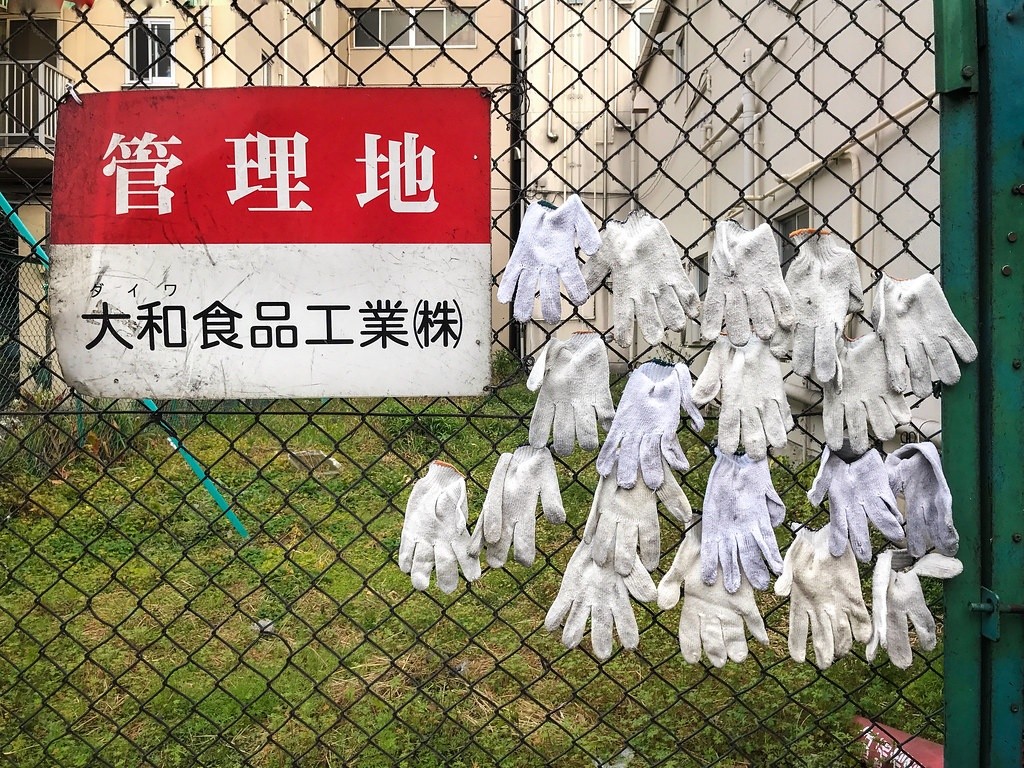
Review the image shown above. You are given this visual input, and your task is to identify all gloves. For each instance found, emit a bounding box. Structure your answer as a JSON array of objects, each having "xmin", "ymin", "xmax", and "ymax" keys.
[{"xmin": 398, "ymin": 194, "xmax": 978, "ymax": 671}]
